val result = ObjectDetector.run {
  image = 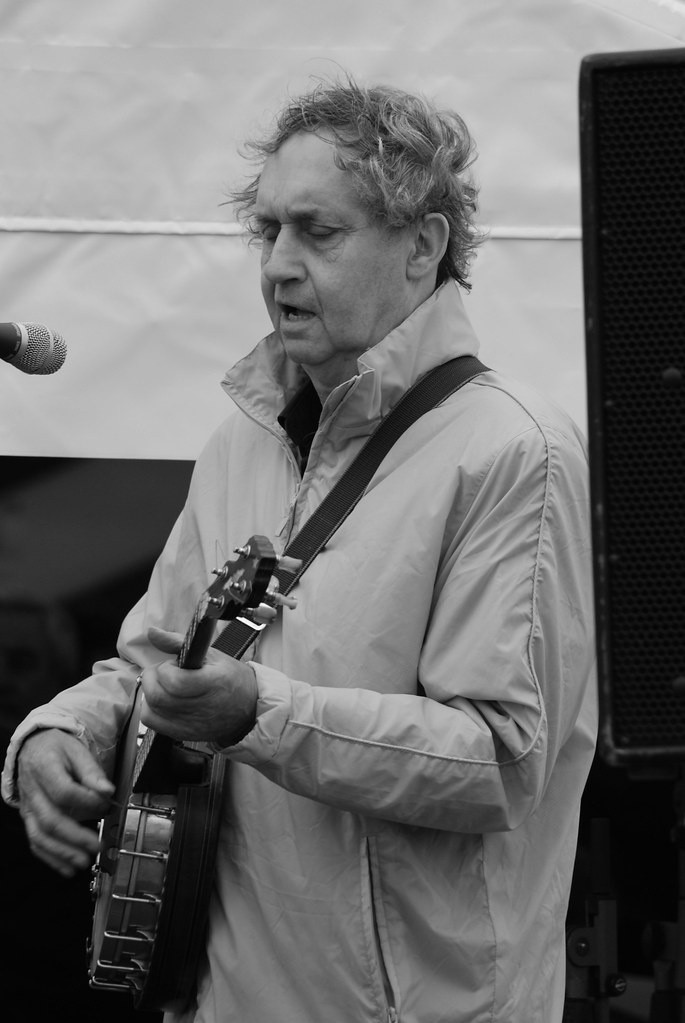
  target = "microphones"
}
[0,322,68,375]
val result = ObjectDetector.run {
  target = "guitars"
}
[86,536,302,1010]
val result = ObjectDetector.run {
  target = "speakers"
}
[579,48,685,769]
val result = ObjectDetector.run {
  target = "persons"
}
[1,70,601,1022]
